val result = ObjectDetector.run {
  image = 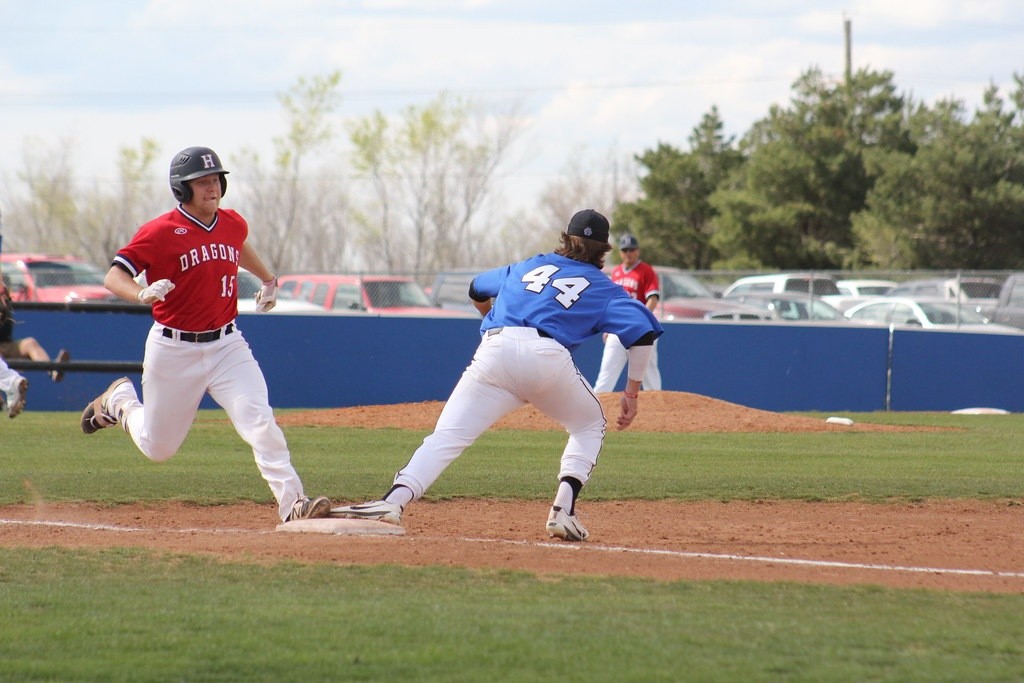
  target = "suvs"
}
[1,252,124,302]
[277,275,469,319]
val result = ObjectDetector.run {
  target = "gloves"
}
[255,275,277,312]
[138,279,176,305]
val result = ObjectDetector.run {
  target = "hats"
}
[618,234,639,249]
[567,209,610,243]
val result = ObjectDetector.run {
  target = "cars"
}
[133,265,326,312]
[724,273,1024,333]
[601,263,783,320]
[431,269,492,318]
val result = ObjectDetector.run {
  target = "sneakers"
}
[329,499,403,526]
[287,496,331,521]
[7,377,28,419]
[52,350,68,382]
[80,376,133,434]
[545,506,588,540]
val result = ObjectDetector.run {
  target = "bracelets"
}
[260,275,276,286]
[623,390,638,398]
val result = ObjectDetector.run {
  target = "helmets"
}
[169,146,230,203]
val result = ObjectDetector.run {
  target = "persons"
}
[0,282,68,418]
[81,147,332,522]
[327,209,664,545]
[593,232,662,393]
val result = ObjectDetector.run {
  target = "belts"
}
[160,324,234,343]
